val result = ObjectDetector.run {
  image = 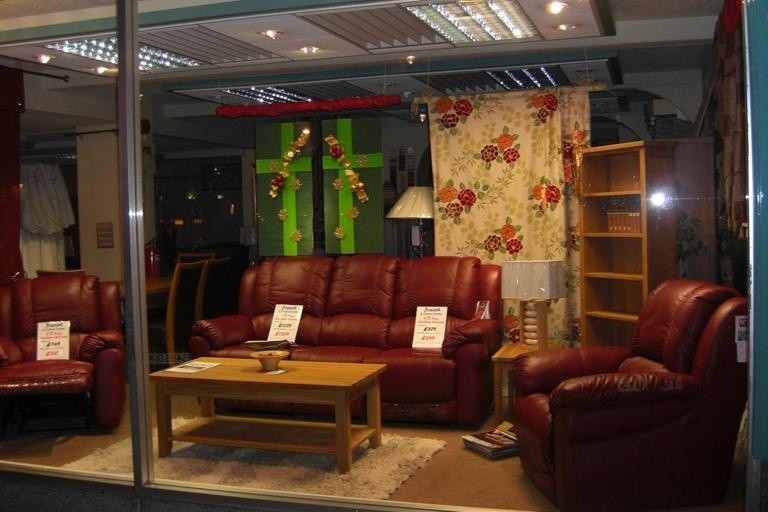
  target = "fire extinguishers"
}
[145,235,160,282]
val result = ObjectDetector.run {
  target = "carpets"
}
[57,415,448,502]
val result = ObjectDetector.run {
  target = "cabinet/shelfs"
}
[578,138,679,347]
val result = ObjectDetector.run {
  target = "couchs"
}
[187,254,505,431]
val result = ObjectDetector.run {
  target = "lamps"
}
[500,260,567,347]
[383,185,434,257]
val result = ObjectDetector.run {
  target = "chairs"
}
[509,276,749,512]
[148,259,210,369]
[176,252,216,262]
[0,271,127,431]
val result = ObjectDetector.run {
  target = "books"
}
[606,202,641,232]
[462,426,519,461]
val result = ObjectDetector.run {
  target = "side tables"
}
[118,275,172,351]
[490,341,561,418]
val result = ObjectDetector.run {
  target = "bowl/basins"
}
[249,350,289,371]
